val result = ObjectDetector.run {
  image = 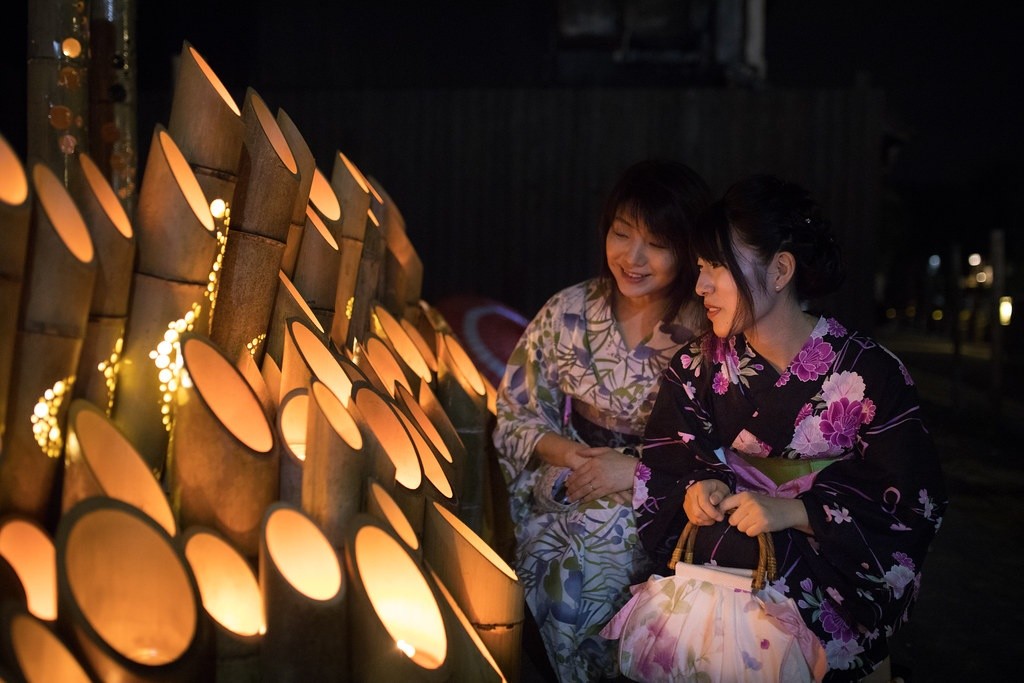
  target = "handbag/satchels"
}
[597,503,830,683]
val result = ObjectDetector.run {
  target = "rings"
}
[589,482,596,491]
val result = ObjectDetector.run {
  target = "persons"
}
[633,172,949,683]
[491,158,713,683]
